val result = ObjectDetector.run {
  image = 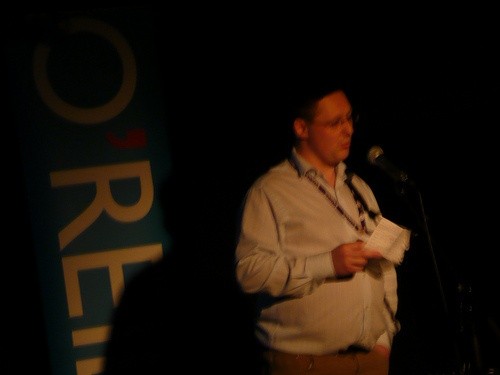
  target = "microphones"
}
[367,146,417,189]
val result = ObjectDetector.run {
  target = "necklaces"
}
[287,152,374,237]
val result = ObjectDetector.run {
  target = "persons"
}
[236,85,400,375]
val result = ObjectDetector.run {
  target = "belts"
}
[338,344,369,355]
